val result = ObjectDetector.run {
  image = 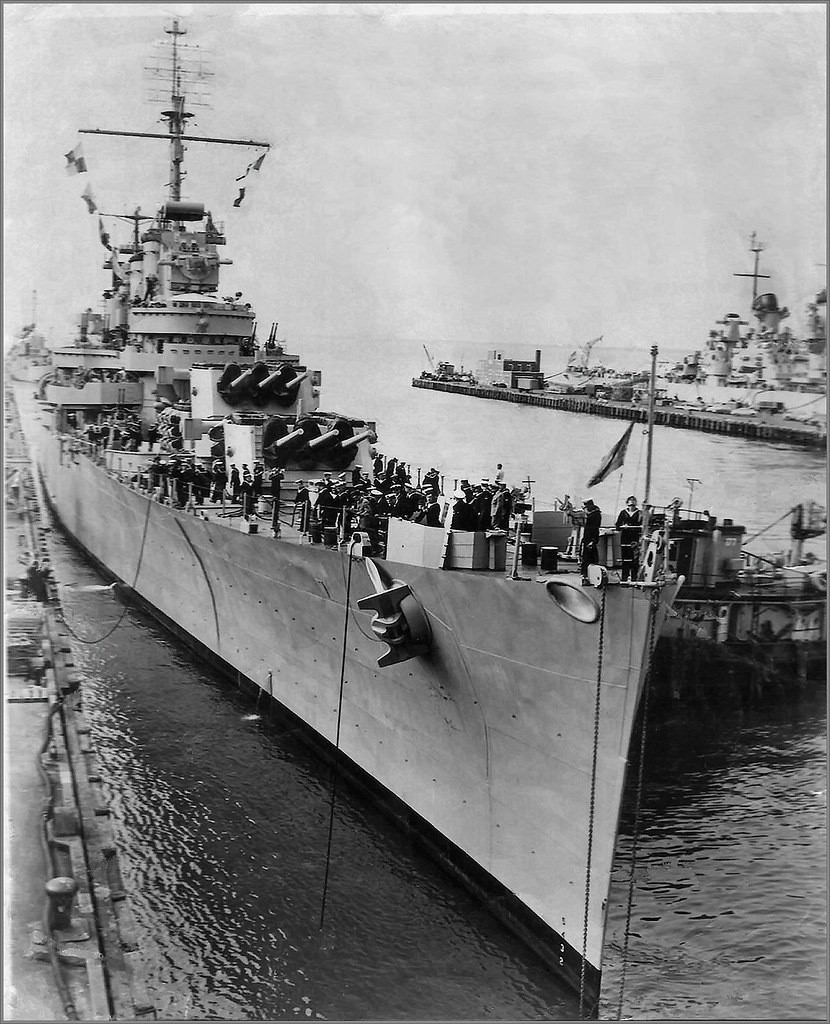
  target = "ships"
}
[1,19,692,1023]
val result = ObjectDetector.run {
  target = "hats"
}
[88,415,140,435]
[148,453,280,478]
[583,499,594,505]
[379,453,406,464]
[292,464,439,499]
[461,478,507,491]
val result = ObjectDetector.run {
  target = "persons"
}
[83,412,159,452]
[293,454,445,551]
[450,464,512,537]
[143,276,155,302]
[580,501,602,587]
[144,453,284,520]
[615,497,644,582]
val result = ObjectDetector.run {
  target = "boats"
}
[635,233,829,420]
[549,360,638,394]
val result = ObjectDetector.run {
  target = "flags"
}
[64,150,114,251]
[587,415,638,488]
[233,154,266,208]
